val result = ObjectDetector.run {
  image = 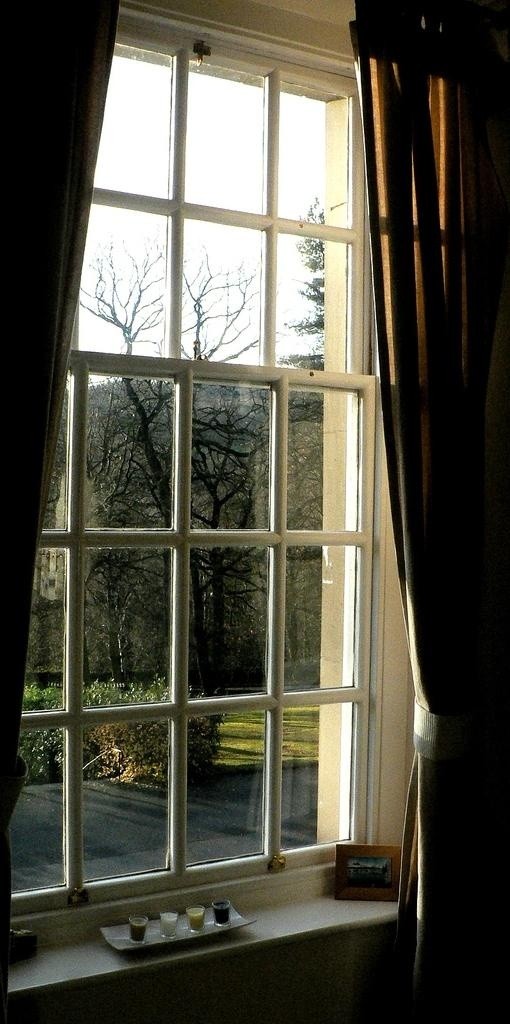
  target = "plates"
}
[98,904,258,952]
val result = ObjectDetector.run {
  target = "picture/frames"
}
[334,842,402,902]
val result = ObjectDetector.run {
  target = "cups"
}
[128,914,149,944]
[159,910,179,938]
[211,898,232,928]
[185,904,207,933]
